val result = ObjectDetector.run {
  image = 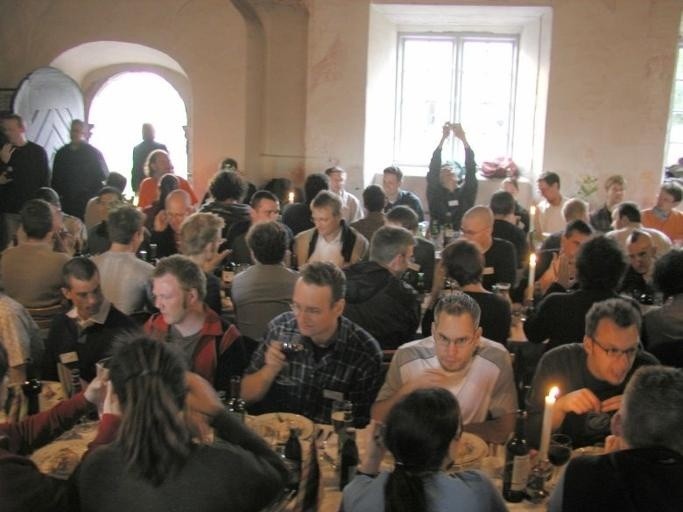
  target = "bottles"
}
[284,430,302,489]
[221,253,235,298]
[149,244,157,265]
[339,429,360,490]
[430,209,440,250]
[69,368,82,398]
[20,357,43,418]
[533,281,544,307]
[226,376,246,425]
[502,409,532,503]
[289,252,299,272]
[442,212,453,248]
[415,273,425,303]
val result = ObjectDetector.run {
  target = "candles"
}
[287,192,295,204]
[527,254,537,299]
[539,396,557,462]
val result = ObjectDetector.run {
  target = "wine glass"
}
[277,333,303,386]
[331,400,353,433]
[547,434,573,490]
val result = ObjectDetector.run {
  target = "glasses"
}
[590,336,644,360]
[457,227,489,238]
[406,254,417,266]
[285,300,324,318]
[654,192,672,205]
[433,328,477,348]
[625,250,648,265]
[308,217,329,224]
[164,209,187,221]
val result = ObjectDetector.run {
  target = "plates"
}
[252,411,314,445]
[458,431,487,463]
[33,439,85,479]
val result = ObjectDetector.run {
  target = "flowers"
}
[574,174,598,195]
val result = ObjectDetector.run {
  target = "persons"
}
[528,171,571,242]
[426,121,478,232]
[382,167,425,223]
[325,166,364,223]
[72,335,293,512]
[200,158,258,205]
[281,172,330,237]
[517,219,592,304]
[461,206,517,293]
[142,255,250,402]
[86,186,151,254]
[0,287,48,393]
[0,198,73,323]
[524,300,661,455]
[50,119,110,222]
[339,386,510,512]
[421,240,512,348]
[616,228,661,304]
[641,182,683,243]
[0,114,50,217]
[85,208,157,323]
[150,189,195,254]
[44,257,138,385]
[228,190,296,268]
[489,191,530,269]
[589,175,627,233]
[640,249,682,367]
[384,205,435,293]
[197,170,256,259]
[546,366,683,512]
[175,212,233,315]
[84,172,127,239]
[340,225,422,349]
[603,202,673,264]
[370,293,518,446]
[230,223,302,342]
[137,149,199,208]
[265,177,295,224]
[240,261,384,429]
[349,184,389,241]
[523,235,642,350]
[0,362,124,512]
[539,198,591,263]
[141,174,180,229]
[131,123,170,197]
[293,191,369,275]
[5,186,83,261]
[493,176,529,235]
